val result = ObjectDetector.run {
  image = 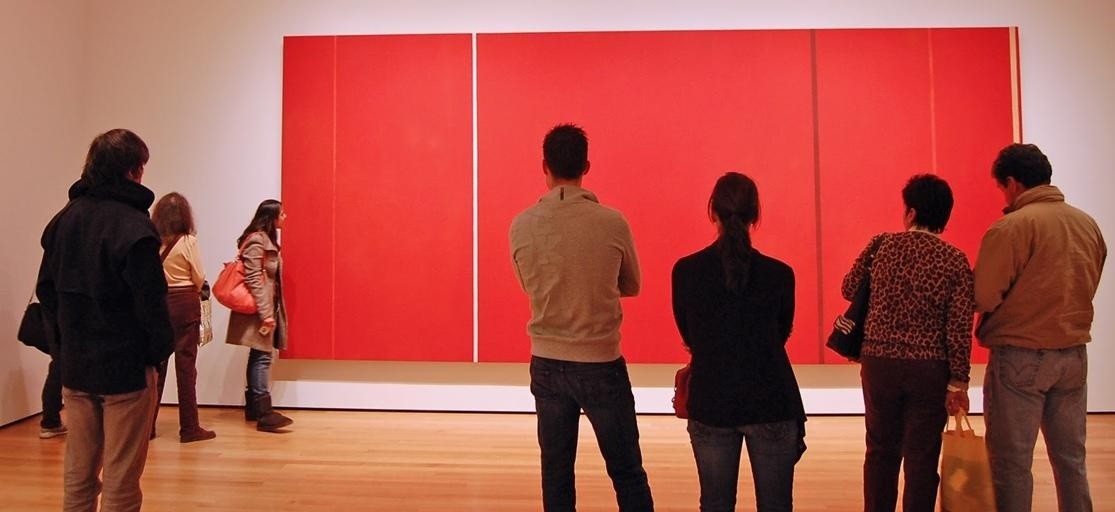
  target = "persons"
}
[971,142,1109,512]
[505,120,656,511]
[224,198,293,431]
[672,172,810,511]
[149,191,216,445]
[33,128,175,512]
[38,357,69,440]
[841,172,976,512]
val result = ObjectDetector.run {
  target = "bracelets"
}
[946,384,968,393]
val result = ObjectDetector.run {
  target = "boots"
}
[255,394,293,430]
[244,391,282,421]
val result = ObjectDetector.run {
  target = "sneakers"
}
[39,426,67,439]
[180,428,215,442]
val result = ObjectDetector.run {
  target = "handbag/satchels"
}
[939,408,997,512]
[671,366,689,419]
[198,299,213,347]
[826,234,880,362]
[213,231,268,315]
[18,301,51,353]
